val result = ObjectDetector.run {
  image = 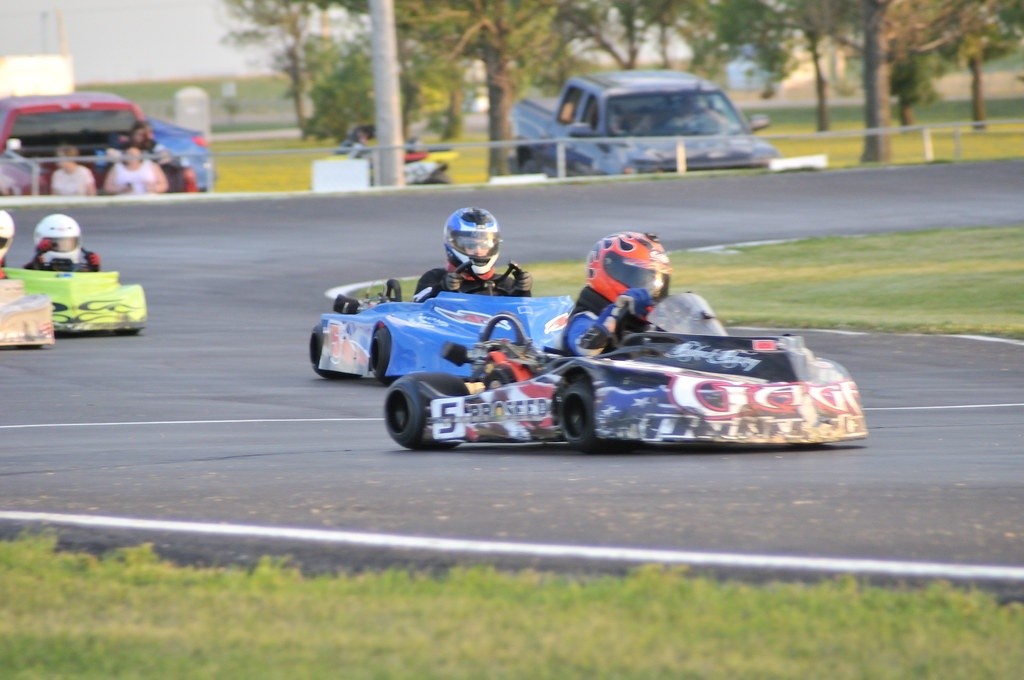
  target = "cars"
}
[0,91,215,194]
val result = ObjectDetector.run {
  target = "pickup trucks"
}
[512,68,780,175]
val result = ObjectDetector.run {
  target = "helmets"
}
[587,232,671,323]
[0,210,14,261]
[443,207,501,274]
[33,213,81,264]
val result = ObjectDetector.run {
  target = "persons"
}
[105,121,171,166]
[562,232,699,362]
[23,213,100,271]
[101,146,169,195]
[50,144,96,196]
[335,129,372,159]
[413,206,533,303]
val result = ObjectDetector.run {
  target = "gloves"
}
[440,272,462,290]
[614,288,655,315]
[512,271,532,291]
